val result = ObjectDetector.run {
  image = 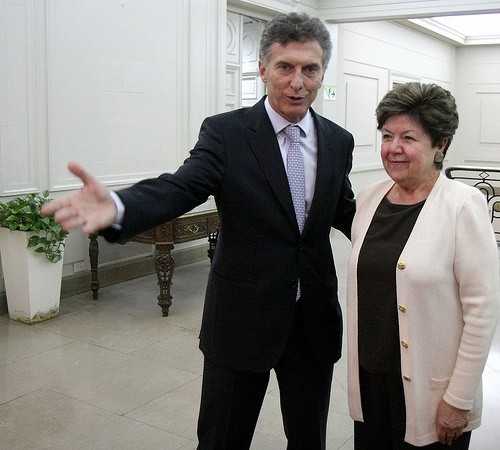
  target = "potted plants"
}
[0,186,72,327]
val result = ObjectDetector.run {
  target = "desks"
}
[88,194,222,317]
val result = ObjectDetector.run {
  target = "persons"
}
[40,12,357,450]
[346,82,500,450]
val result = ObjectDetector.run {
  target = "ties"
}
[281,126,306,305]
